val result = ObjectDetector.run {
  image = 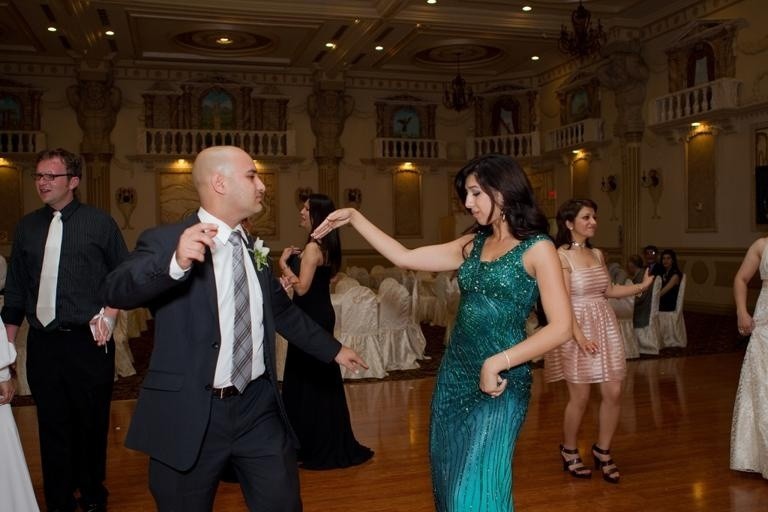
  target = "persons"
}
[278,195,374,471]
[656,249,682,311]
[543,197,655,486]
[642,244,663,276]
[726,235,767,481]
[626,252,653,328]
[0,147,129,511]
[0,308,42,511]
[97,146,367,512]
[310,153,572,512]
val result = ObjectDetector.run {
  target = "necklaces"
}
[570,243,589,248]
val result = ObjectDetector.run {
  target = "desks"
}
[13,307,153,396]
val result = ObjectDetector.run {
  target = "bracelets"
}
[634,282,642,298]
[502,351,511,371]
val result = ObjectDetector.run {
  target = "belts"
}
[211,386,244,396]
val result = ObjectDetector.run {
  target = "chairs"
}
[658,273,688,349]
[433,273,449,327]
[613,279,641,362]
[635,275,663,355]
[444,277,462,345]
[330,258,643,295]
[334,285,389,379]
[526,310,546,362]
[416,268,435,324]
[406,280,433,360]
[376,283,421,371]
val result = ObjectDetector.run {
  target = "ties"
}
[228,231,252,395]
[36,211,63,328]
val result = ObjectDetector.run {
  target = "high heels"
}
[591,443,620,482]
[559,444,592,477]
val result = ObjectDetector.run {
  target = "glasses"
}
[32,172,71,182]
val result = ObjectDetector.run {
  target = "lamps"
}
[441,54,475,111]
[299,188,311,203]
[118,187,134,203]
[348,189,357,202]
[641,169,659,189]
[600,175,617,193]
[558,0,607,65]
